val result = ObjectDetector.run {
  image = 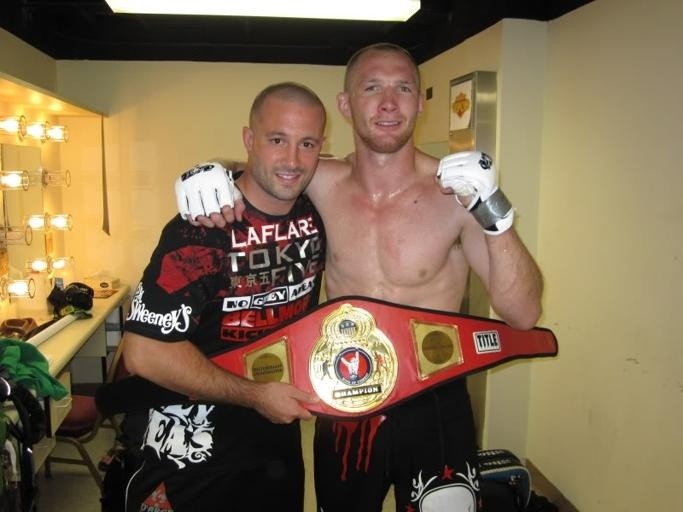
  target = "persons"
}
[99,80,326,510]
[172,42,546,512]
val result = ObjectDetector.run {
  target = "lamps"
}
[103,0,422,23]
[0,114,74,304]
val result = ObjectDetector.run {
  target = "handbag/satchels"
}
[477,449,531,508]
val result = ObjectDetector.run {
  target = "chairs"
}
[45,330,126,494]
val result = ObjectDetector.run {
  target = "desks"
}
[0,285,132,512]
